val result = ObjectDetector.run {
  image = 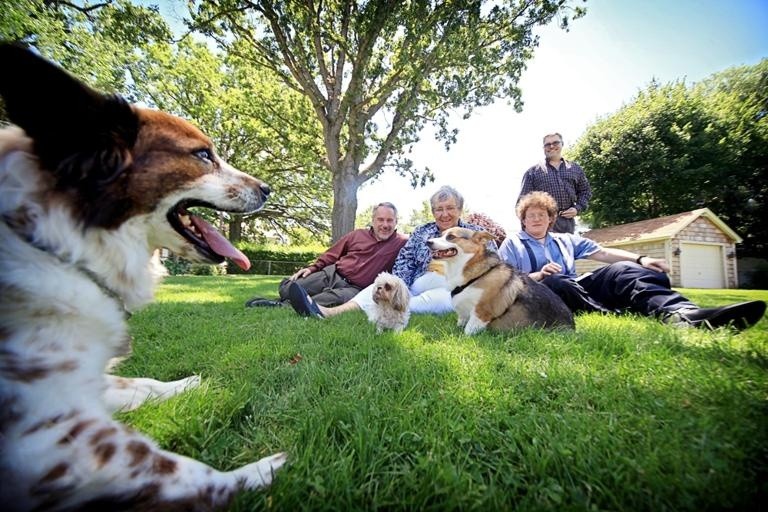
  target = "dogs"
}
[0,39,288,512]
[366,270,411,336]
[426,227,576,337]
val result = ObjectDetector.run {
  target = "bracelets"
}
[636,255,646,264]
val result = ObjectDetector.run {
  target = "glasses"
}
[544,141,561,148]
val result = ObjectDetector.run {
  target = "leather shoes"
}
[663,300,766,336]
[247,282,325,320]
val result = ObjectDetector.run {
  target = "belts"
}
[335,271,352,284]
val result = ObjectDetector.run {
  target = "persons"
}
[496,191,766,335]
[247,201,409,309]
[516,133,591,234]
[289,185,499,320]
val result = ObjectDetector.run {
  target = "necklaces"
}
[531,236,546,240]
